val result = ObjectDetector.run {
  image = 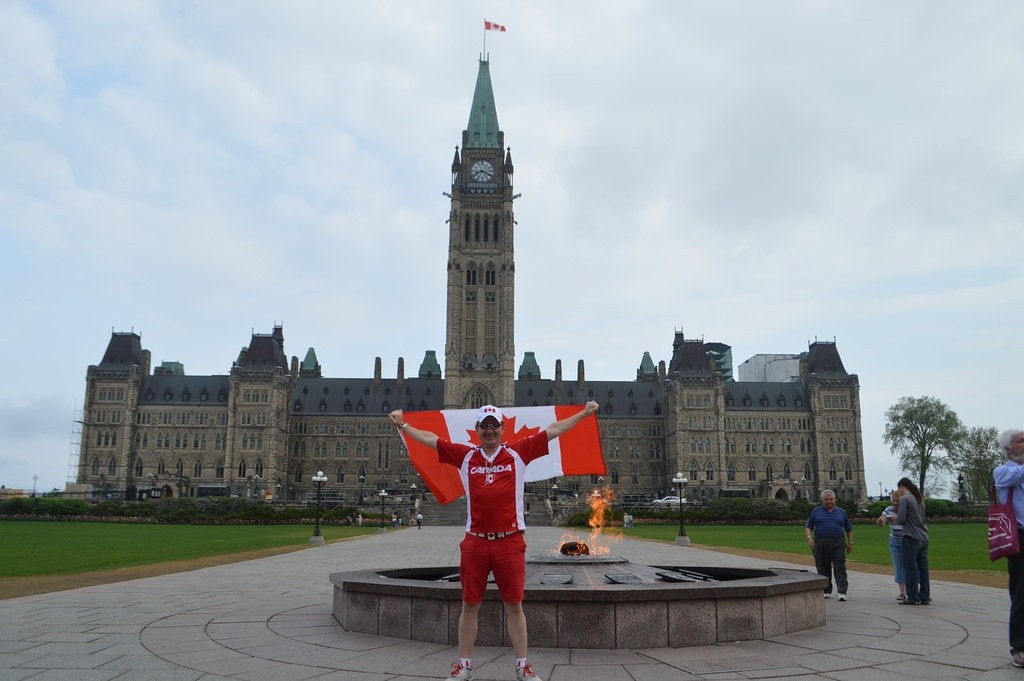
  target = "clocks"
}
[470,159,496,184]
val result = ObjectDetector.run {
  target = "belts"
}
[467,530,516,540]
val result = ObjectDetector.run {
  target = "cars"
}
[550,487,580,498]
[652,496,688,504]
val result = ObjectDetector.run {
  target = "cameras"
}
[886,515,892,519]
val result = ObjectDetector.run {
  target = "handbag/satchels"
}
[987,479,1020,562]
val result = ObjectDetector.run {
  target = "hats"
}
[478,405,503,423]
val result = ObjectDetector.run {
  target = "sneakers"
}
[515,663,542,681]
[445,662,474,681]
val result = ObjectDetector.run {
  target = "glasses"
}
[477,423,501,430]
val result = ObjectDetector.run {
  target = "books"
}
[886,515,893,523]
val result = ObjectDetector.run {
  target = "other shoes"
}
[897,594,906,600]
[1009,651,1024,667]
[838,593,847,601]
[823,593,829,598]
[919,600,929,605]
[899,598,920,605]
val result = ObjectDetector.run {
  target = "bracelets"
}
[398,421,409,431]
[807,535,811,539]
[848,541,854,545]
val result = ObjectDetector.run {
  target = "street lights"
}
[379,490,388,529]
[32,474,39,497]
[557,480,561,495]
[800,476,807,496]
[672,473,688,537]
[409,483,417,518]
[311,471,328,536]
[359,475,366,499]
[147,473,153,490]
[253,475,259,495]
[878,481,884,501]
[277,484,281,500]
[599,476,604,492]
[417,472,421,492]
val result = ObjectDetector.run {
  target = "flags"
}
[393,404,608,507]
[485,21,506,32]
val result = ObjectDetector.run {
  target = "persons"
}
[260,487,270,501]
[398,516,402,527]
[805,489,854,601]
[993,427,1024,667]
[876,492,913,600]
[392,512,397,527]
[387,400,600,681]
[892,477,930,605]
[958,474,966,489]
[417,512,423,529]
